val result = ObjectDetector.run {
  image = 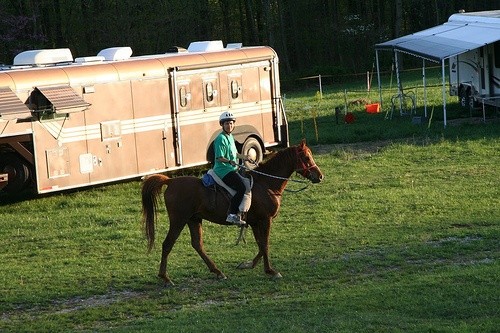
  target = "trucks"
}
[0,38,289,194]
[448,9,500,113]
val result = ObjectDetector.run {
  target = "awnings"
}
[374,22,500,128]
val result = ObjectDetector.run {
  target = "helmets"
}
[219,112,235,124]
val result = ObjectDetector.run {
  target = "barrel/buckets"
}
[364,102,380,113]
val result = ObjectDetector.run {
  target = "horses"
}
[140,137,325,290]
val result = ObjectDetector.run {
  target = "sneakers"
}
[226,215,246,224]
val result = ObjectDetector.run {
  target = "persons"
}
[213,112,251,225]
